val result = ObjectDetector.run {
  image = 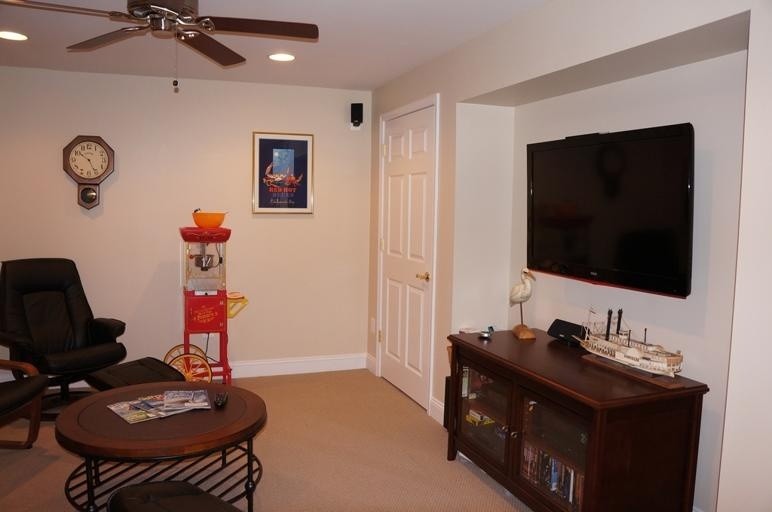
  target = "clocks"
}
[62,135,115,210]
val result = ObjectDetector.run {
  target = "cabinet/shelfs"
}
[446,328,709,512]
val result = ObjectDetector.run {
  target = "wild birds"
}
[509,267,536,307]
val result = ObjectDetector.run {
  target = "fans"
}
[0,0,318,66]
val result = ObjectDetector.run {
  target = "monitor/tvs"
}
[526,123,694,298]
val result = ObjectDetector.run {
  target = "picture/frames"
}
[252,131,314,215]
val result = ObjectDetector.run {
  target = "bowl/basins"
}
[191,211,227,228]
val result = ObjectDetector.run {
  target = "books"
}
[465,408,584,510]
[163,390,195,410]
[185,389,214,410]
[105,400,150,426]
[129,402,164,420]
[137,394,181,412]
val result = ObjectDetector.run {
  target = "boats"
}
[572,304,684,378]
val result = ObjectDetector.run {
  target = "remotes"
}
[214,393,227,407]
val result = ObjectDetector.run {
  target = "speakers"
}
[351,103,363,126]
[547,319,591,344]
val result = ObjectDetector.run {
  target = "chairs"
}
[0,258,186,449]
